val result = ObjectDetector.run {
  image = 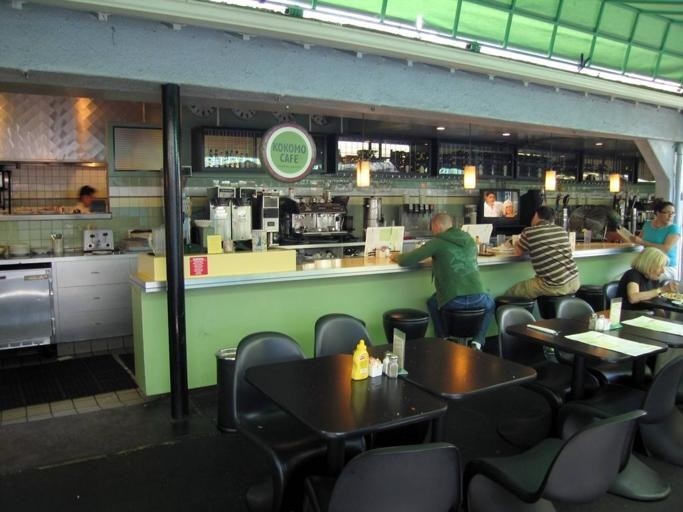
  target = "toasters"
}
[82,228,116,253]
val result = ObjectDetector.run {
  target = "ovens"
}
[0,262,57,369]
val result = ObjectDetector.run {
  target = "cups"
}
[583,230,591,243]
[568,231,576,249]
[223,239,234,252]
[497,234,506,246]
[52,239,63,255]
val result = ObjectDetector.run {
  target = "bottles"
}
[350,339,399,381]
[350,378,368,424]
[322,191,330,203]
[287,188,294,200]
[442,144,637,185]
[588,313,610,332]
[205,149,253,169]
[474,235,487,254]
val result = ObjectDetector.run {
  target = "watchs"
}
[655,287,662,297]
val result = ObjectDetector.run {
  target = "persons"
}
[615,247,679,371]
[73,185,97,214]
[505,205,581,301]
[484,191,500,217]
[629,201,681,318]
[502,199,517,218]
[389,212,496,351]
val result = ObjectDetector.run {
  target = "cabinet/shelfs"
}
[190,126,335,174]
[51,258,136,342]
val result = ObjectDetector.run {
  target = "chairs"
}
[302,444,463,511]
[552,296,653,388]
[464,403,646,511]
[604,283,619,311]
[313,313,434,444]
[231,332,365,510]
[495,304,598,404]
[567,357,679,442]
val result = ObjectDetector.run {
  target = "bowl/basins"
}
[194,219,211,227]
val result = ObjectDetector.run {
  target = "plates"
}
[8,243,31,257]
[29,247,49,257]
[660,293,683,302]
[492,247,515,251]
[15,210,34,215]
[36,210,58,215]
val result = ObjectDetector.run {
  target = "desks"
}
[245,354,449,469]
[505,317,668,399]
[572,307,682,388]
[640,296,682,314]
[351,336,538,444]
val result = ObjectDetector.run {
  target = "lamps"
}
[464,122,476,189]
[356,115,370,187]
[609,139,621,192]
[544,132,556,190]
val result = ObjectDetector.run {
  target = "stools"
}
[579,285,604,301]
[445,306,485,342]
[493,295,536,325]
[383,308,429,343]
[538,293,574,318]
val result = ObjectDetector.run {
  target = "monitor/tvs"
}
[461,224,493,253]
[480,188,521,225]
[363,226,404,257]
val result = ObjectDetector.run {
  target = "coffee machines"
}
[280,194,356,244]
[617,199,656,236]
